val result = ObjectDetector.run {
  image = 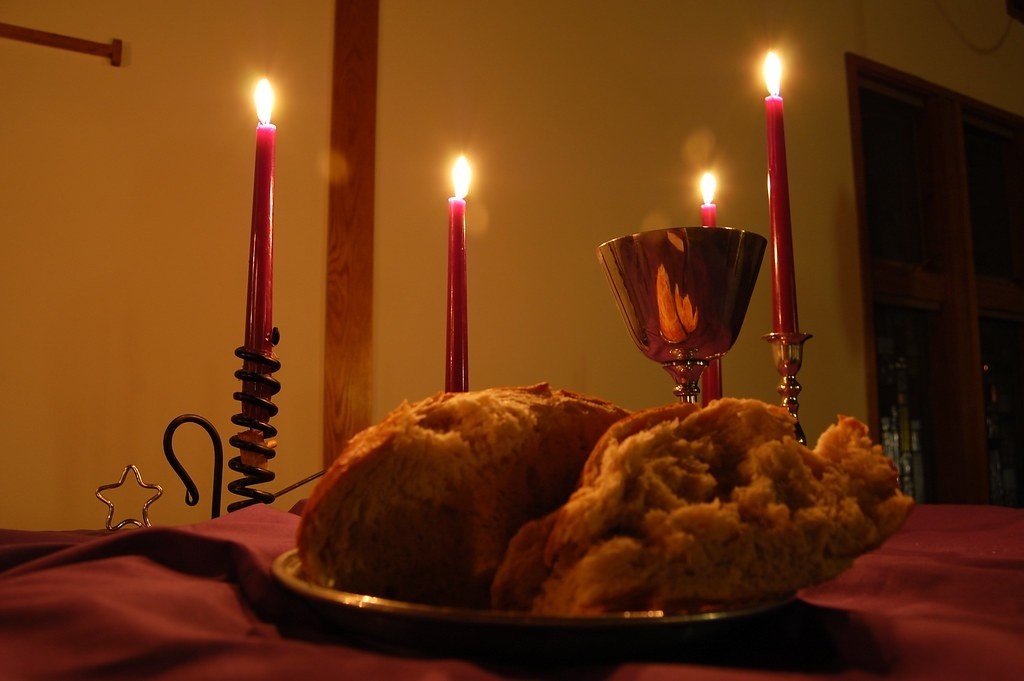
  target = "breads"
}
[297,382,643,601]
[490,393,913,612]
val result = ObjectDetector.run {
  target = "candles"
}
[445,197,469,395]
[702,204,722,409]
[237,124,276,471]
[764,94,798,334]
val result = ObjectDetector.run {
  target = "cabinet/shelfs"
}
[844,51,1024,508]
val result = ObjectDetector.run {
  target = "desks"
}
[0,502,1024,681]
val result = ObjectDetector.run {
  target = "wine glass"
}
[594,226,769,405]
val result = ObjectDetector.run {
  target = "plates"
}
[270,548,796,632]
